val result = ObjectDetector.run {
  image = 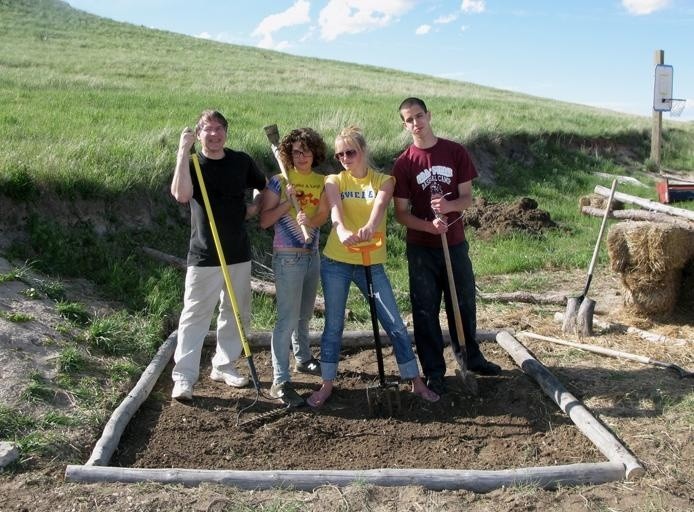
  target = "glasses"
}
[291,149,313,158]
[334,149,357,160]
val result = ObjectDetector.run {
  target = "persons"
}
[259,128,330,406]
[391,98,502,395]
[171,109,267,402]
[306,123,440,407]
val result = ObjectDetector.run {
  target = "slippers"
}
[411,388,440,402]
[306,390,333,407]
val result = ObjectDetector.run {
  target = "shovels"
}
[562,178,617,337]
[441,233,484,397]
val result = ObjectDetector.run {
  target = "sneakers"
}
[210,364,249,387]
[295,355,322,377]
[427,372,444,394]
[270,380,305,406]
[468,355,501,376]
[171,380,194,400]
